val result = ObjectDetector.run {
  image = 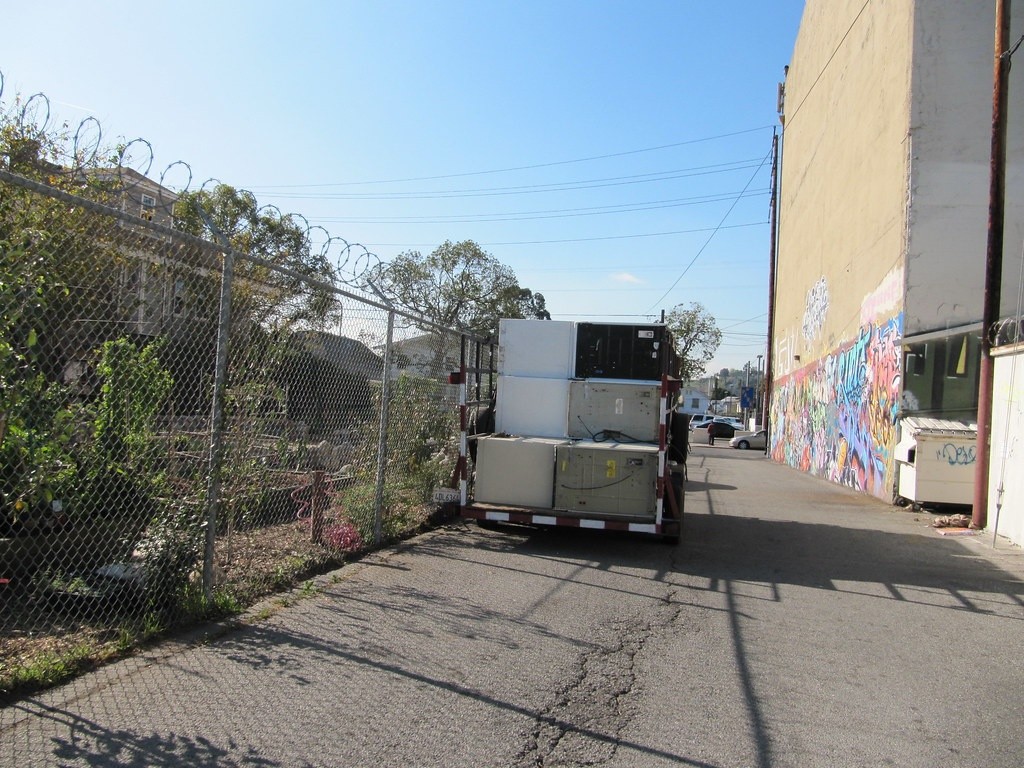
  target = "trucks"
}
[462,310,688,547]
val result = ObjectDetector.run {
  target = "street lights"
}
[756,354,764,424]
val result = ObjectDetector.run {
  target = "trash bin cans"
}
[894,416,977,505]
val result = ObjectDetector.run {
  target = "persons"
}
[707,421,716,446]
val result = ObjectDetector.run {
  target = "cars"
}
[729,428,767,450]
[689,414,744,438]
